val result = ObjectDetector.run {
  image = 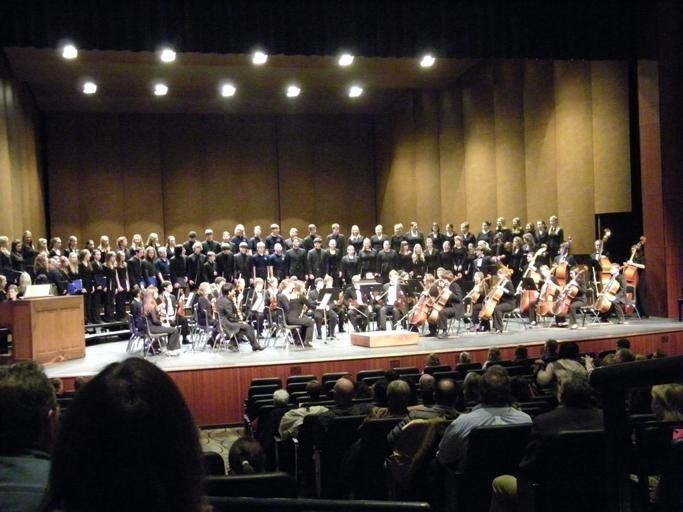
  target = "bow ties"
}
[596,252,599,254]
[478,256,483,259]
[389,284,394,286]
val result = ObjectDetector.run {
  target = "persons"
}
[547,216,563,261]
[102,252,118,320]
[400,273,414,298]
[404,221,425,251]
[630,245,651,320]
[276,279,291,324]
[234,278,251,309]
[615,338,630,353]
[326,240,343,288]
[489,376,616,511]
[142,249,158,289]
[425,355,440,367]
[187,242,207,290]
[324,276,346,332]
[444,224,457,249]
[650,382,683,424]
[130,234,144,255]
[307,277,338,340]
[0,235,15,284]
[553,242,576,270]
[424,238,439,275]
[48,237,64,258]
[62,235,81,257]
[452,235,467,275]
[79,249,96,324]
[510,217,525,239]
[258,389,297,450]
[6,285,21,302]
[228,436,265,476]
[233,242,256,287]
[251,242,272,285]
[358,238,377,278]
[146,232,160,255]
[128,248,144,289]
[511,236,523,286]
[460,223,476,249]
[347,224,365,255]
[534,342,589,405]
[0,357,63,512]
[217,231,236,255]
[384,378,462,475]
[21,229,39,278]
[156,281,192,344]
[478,240,495,258]
[43,358,213,511]
[473,246,492,281]
[476,269,517,335]
[466,242,476,270]
[362,380,411,421]
[326,224,346,254]
[590,241,604,263]
[98,235,110,263]
[201,229,221,256]
[307,238,330,282]
[462,272,487,331]
[529,264,568,326]
[266,279,279,322]
[377,270,400,330]
[371,224,386,251]
[439,241,454,271]
[344,274,370,332]
[384,369,400,383]
[424,270,465,339]
[131,288,161,355]
[537,221,548,244]
[250,226,267,253]
[557,267,587,329]
[36,274,48,286]
[287,280,316,347]
[208,277,227,302]
[143,285,180,356]
[169,245,191,297]
[531,340,560,371]
[427,223,445,253]
[513,346,529,364]
[596,262,626,324]
[42,256,72,294]
[408,273,436,332]
[247,278,270,339]
[266,224,287,255]
[115,251,131,317]
[19,274,31,296]
[461,372,486,416]
[0,276,9,303]
[355,381,371,398]
[476,222,494,248]
[521,253,545,295]
[69,252,87,295]
[340,245,361,285]
[375,238,400,285]
[231,223,250,253]
[503,241,513,263]
[482,347,502,372]
[202,252,218,283]
[584,349,636,379]
[525,222,537,243]
[197,282,223,349]
[392,223,407,253]
[182,231,201,255]
[403,377,419,408]
[652,350,667,361]
[166,236,177,259]
[115,236,132,259]
[436,267,447,280]
[511,377,552,421]
[412,244,428,277]
[49,379,64,397]
[216,243,234,283]
[285,227,304,251]
[399,241,413,272]
[10,240,24,272]
[303,224,323,251]
[286,237,308,282]
[521,233,538,264]
[215,283,266,351]
[420,371,531,512]
[315,377,375,451]
[495,217,512,242]
[269,243,287,285]
[459,352,472,365]
[156,246,171,285]
[85,240,96,252]
[37,238,50,255]
[90,249,107,320]
[305,380,322,402]
[360,380,389,416]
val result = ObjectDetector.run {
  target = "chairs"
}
[276,307,305,349]
[393,367,423,382]
[264,306,276,347]
[447,297,478,336]
[626,286,642,322]
[521,357,543,375]
[628,414,683,511]
[580,289,601,328]
[468,423,545,474]
[322,371,353,405]
[244,376,295,443]
[457,362,486,377]
[486,360,523,377]
[286,375,335,407]
[203,473,296,496]
[598,350,617,360]
[357,369,386,388]
[578,351,596,361]
[363,418,405,450]
[501,293,528,332]
[190,302,214,352]
[302,414,366,453]
[423,365,458,381]
[213,311,240,352]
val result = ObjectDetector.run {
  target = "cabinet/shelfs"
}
[8,295,86,364]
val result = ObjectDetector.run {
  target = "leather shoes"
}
[166,351,180,357]
[228,344,239,353]
[493,329,504,334]
[207,338,216,348]
[426,331,438,337]
[529,322,538,328]
[316,335,323,340]
[304,341,313,347]
[466,322,471,329]
[182,339,193,344]
[148,348,160,355]
[340,330,347,332]
[253,346,267,352]
[271,332,276,337]
[295,339,303,345]
[257,334,265,339]
[328,334,337,340]
[570,324,579,330]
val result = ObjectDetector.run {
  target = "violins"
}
[177,290,186,316]
[338,290,368,320]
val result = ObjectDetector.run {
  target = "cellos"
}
[592,266,623,313]
[555,234,574,285]
[478,261,509,321]
[515,243,547,315]
[535,263,557,316]
[462,272,491,303]
[408,292,436,327]
[549,266,588,316]
[597,227,613,291]
[420,273,462,325]
[623,235,648,317]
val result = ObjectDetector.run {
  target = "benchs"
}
[589,355,683,386]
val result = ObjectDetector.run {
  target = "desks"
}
[126,311,169,359]
